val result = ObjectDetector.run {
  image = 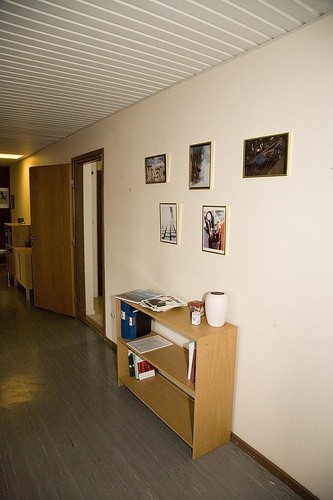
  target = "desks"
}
[13,246,33,302]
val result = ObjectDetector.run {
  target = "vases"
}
[204,291,228,327]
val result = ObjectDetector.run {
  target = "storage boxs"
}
[188,300,205,316]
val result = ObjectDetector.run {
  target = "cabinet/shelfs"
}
[115,294,237,460]
[5,223,31,288]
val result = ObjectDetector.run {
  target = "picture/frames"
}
[201,205,226,255]
[243,132,289,178]
[188,141,212,190]
[159,203,178,245]
[144,154,167,184]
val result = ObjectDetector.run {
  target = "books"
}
[188,341,196,383]
[128,349,156,380]
[127,335,174,355]
[114,288,188,340]
